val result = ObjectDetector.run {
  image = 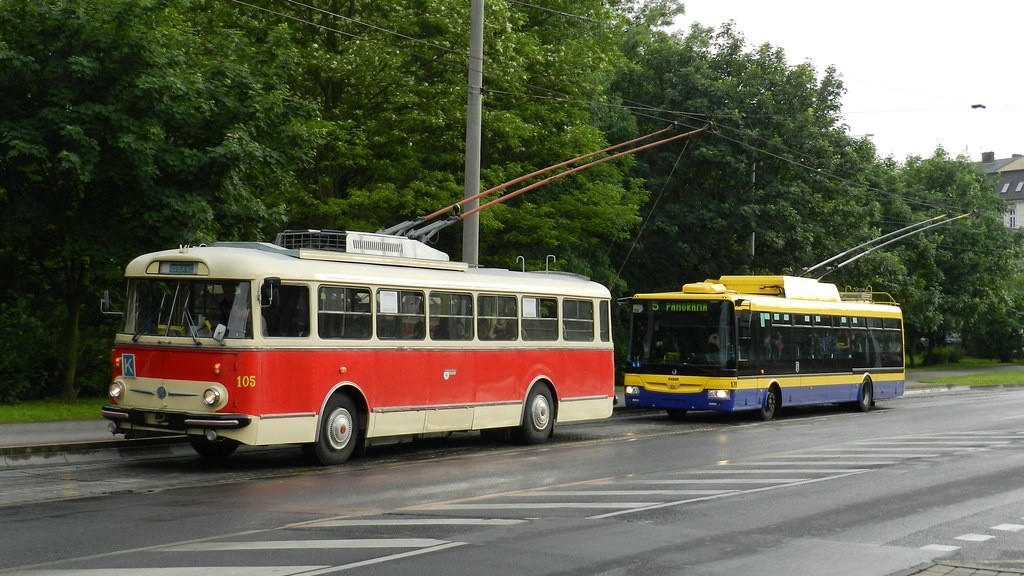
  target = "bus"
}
[615,211,972,422]
[100,120,714,467]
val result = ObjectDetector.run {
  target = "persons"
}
[686,334,731,366]
[411,311,527,339]
[223,293,268,338]
[801,331,901,369]
